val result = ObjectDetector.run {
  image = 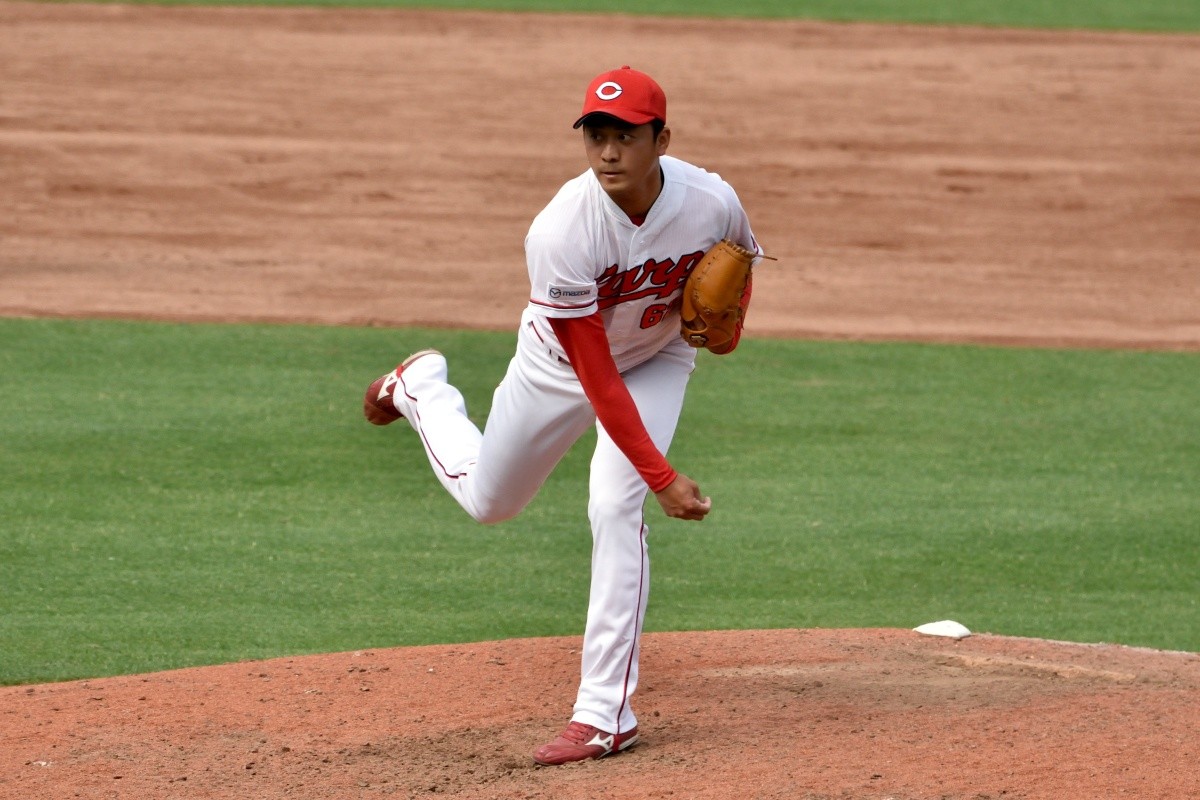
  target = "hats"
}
[573,65,667,128]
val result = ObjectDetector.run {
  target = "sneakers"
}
[362,348,443,425]
[532,720,640,767]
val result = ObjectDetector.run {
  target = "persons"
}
[365,65,765,768]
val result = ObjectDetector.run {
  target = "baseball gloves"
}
[680,238,756,348]
